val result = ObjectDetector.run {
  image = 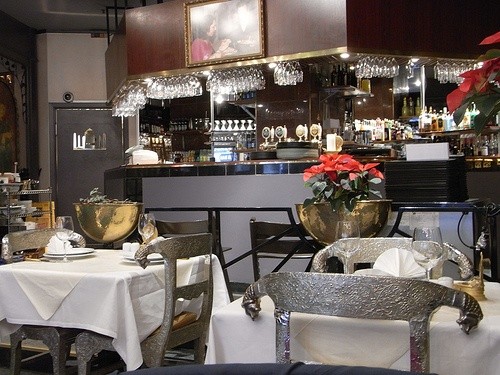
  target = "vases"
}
[295,200,395,245]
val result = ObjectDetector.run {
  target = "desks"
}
[204,279,500,374]
[0,249,230,375]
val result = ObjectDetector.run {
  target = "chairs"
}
[0,217,483,375]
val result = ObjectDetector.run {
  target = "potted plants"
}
[72,187,144,244]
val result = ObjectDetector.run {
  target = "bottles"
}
[340,97,500,157]
[139,118,257,163]
[328,63,362,89]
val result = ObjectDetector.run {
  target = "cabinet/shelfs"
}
[137,72,395,158]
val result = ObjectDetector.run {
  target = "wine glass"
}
[110,73,203,118]
[334,222,361,274]
[434,58,483,85]
[355,54,415,79]
[137,212,156,243]
[55,215,74,264]
[206,65,266,93]
[410,227,445,281]
[273,60,305,86]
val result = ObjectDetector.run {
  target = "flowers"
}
[445,31,500,136]
[302,152,385,215]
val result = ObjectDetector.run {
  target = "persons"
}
[191,15,232,61]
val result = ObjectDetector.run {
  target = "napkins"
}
[49,232,73,251]
[373,248,427,277]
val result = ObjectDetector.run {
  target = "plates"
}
[249,142,318,160]
[43,247,164,264]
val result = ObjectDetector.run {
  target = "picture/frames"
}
[183,0,266,69]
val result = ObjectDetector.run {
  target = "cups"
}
[122,242,140,255]
[439,277,454,288]
